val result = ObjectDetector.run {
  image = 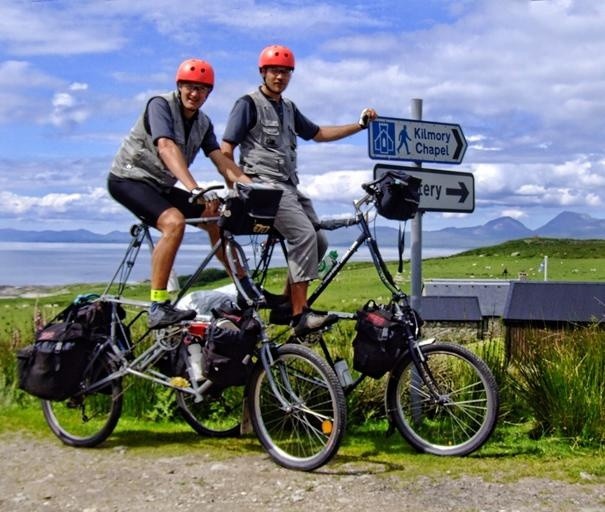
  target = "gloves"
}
[358,108,373,129]
[190,187,220,202]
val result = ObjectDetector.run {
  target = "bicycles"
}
[175,172,500,456]
[40,186,347,468]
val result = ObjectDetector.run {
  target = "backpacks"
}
[16,300,88,402]
[59,292,137,395]
[351,299,421,380]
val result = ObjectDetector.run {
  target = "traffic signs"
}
[373,164,475,213]
[367,117,467,165]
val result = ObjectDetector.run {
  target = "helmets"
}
[258,44,297,71]
[174,57,215,92]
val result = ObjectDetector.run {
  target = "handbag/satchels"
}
[201,298,263,387]
[216,182,285,235]
[372,170,424,220]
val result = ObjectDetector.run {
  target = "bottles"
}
[187,337,210,382]
[334,356,353,387]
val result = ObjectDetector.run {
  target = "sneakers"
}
[236,290,292,311]
[292,311,340,339]
[146,300,197,329]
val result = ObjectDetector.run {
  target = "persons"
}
[106,57,292,328]
[218,44,378,336]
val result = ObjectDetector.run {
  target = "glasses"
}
[180,82,210,93]
